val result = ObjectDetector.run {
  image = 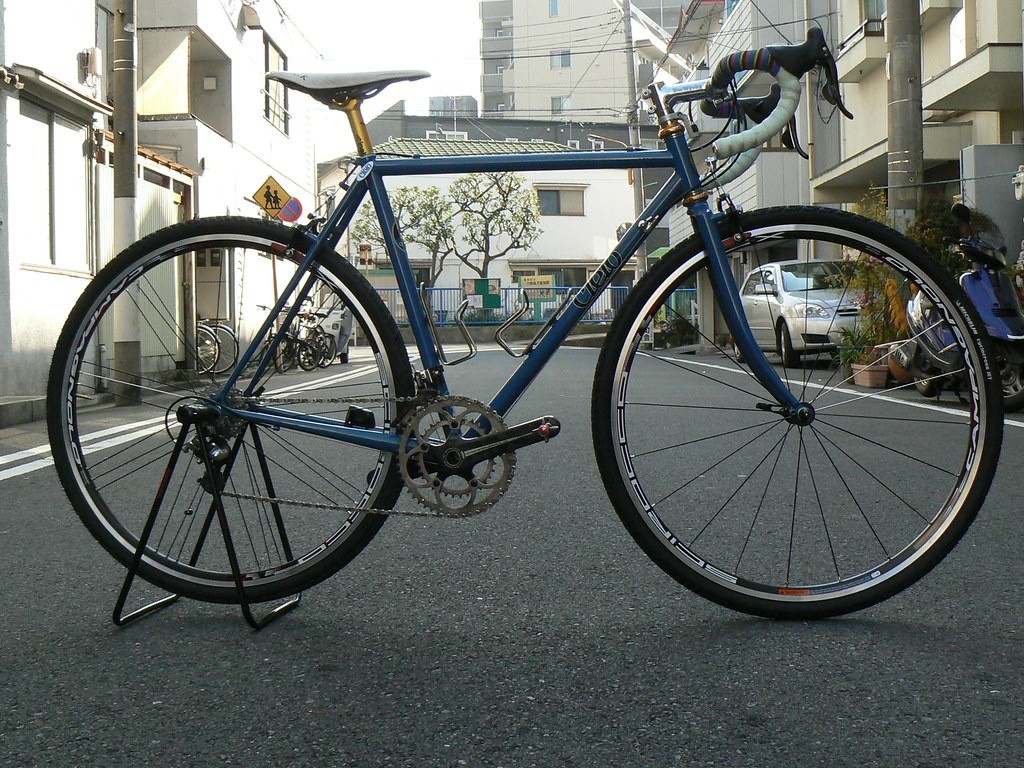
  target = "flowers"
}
[823,192,898,346]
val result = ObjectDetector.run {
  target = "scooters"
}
[898,202,1024,408]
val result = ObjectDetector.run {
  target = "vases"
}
[863,345,875,354]
[888,347,913,381]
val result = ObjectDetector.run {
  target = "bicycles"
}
[257,296,336,374]
[195,317,240,375]
[46,28,1005,631]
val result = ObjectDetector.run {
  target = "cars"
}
[732,259,874,369]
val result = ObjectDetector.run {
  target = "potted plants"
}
[715,335,728,347]
[851,347,891,389]
[830,324,870,383]
[663,311,699,349]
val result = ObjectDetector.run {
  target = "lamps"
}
[82,46,103,78]
